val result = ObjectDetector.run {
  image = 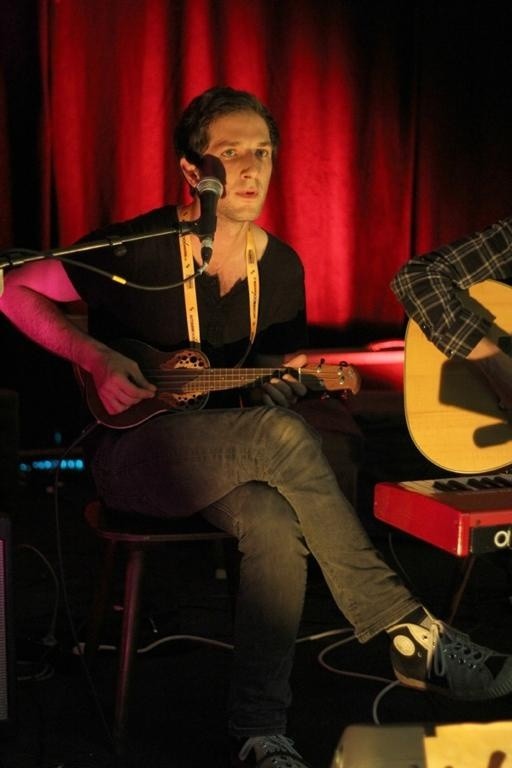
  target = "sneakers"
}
[386,606,512,701]
[238,734,308,768]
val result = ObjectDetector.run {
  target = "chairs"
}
[64,315,243,754]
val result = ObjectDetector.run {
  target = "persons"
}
[2,83,512,766]
[388,203,511,434]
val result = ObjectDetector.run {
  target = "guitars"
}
[75,338,359,431]
[403,280,512,474]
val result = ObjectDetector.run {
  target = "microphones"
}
[197,176,223,265]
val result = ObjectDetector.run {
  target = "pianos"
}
[370,473,510,560]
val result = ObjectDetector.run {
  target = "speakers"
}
[329,724,512,768]
